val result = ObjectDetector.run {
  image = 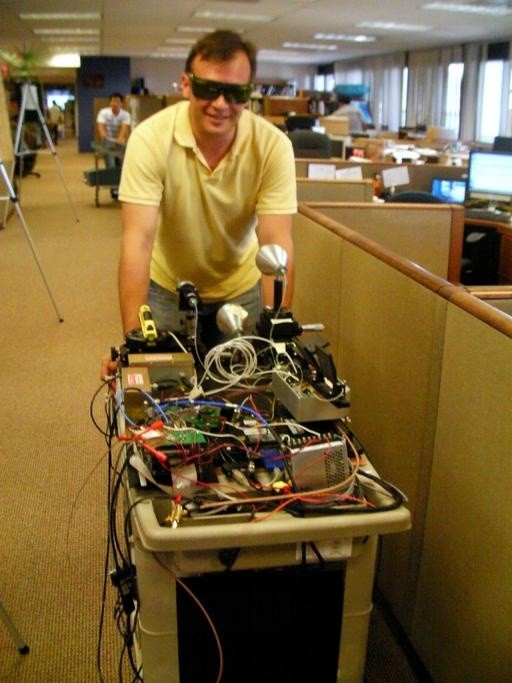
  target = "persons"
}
[96,90,134,199]
[99,26,299,402]
[48,101,64,145]
[329,94,369,132]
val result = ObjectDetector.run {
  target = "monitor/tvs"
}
[430,178,467,204]
[466,149,512,214]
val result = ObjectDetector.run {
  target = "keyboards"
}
[464,210,510,222]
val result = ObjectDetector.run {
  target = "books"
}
[245,82,297,116]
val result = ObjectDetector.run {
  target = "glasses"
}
[187,70,254,104]
